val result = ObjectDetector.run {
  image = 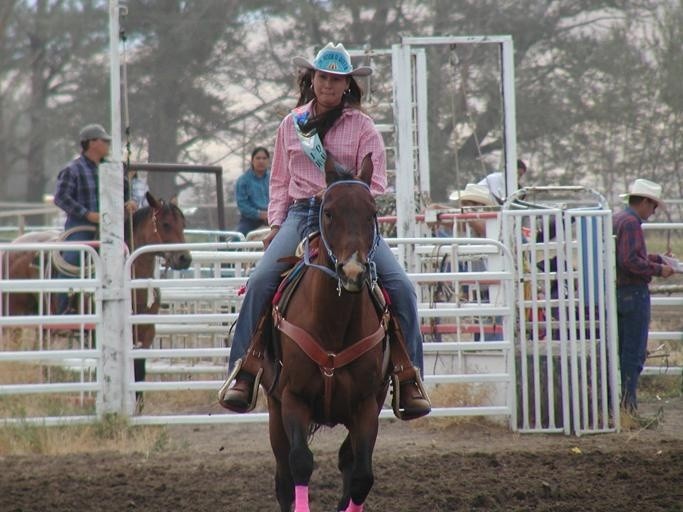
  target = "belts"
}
[293,196,321,205]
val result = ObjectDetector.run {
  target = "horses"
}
[0,191,193,416]
[263,149,392,512]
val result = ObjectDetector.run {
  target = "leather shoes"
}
[223,379,253,409]
[390,384,430,417]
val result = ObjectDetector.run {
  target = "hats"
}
[618,178,666,212]
[292,41,372,76]
[79,124,111,142]
[450,183,495,207]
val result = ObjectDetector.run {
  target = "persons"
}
[477,160,527,207]
[421,182,553,341]
[222,44,433,416]
[230,147,271,245]
[612,179,678,426]
[53,125,151,314]
